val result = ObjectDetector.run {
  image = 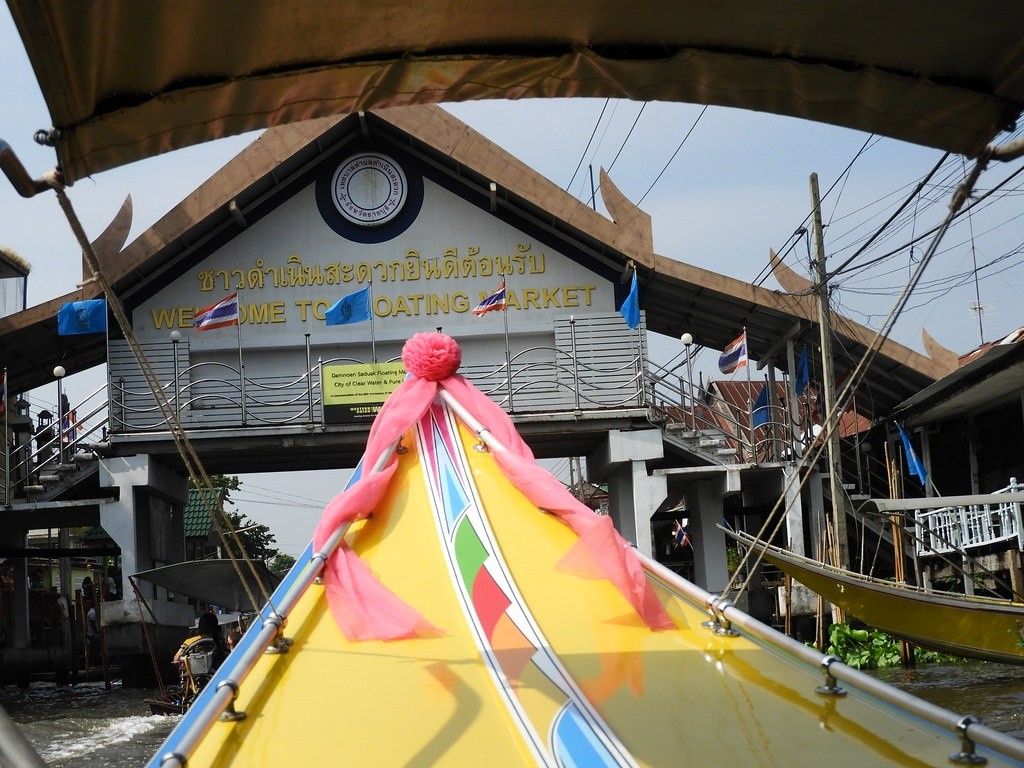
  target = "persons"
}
[107,570,119,600]
[173,613,229,703]
[86,601,100,663]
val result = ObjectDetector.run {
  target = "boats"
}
[128,559,276,716]
[715,489,1024,664]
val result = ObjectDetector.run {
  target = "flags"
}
[324,286,372,326]
[194,292,239,332]
[57,299,106,336]
[619,271,641,333]
[471,282,506,318]
[718,332,748,376]
[674,521,689,547]
[57,411,83,443]
[0,373,7,414]
[896,424,927,485]
[752,383,770,428]
[796,347,810,397]
